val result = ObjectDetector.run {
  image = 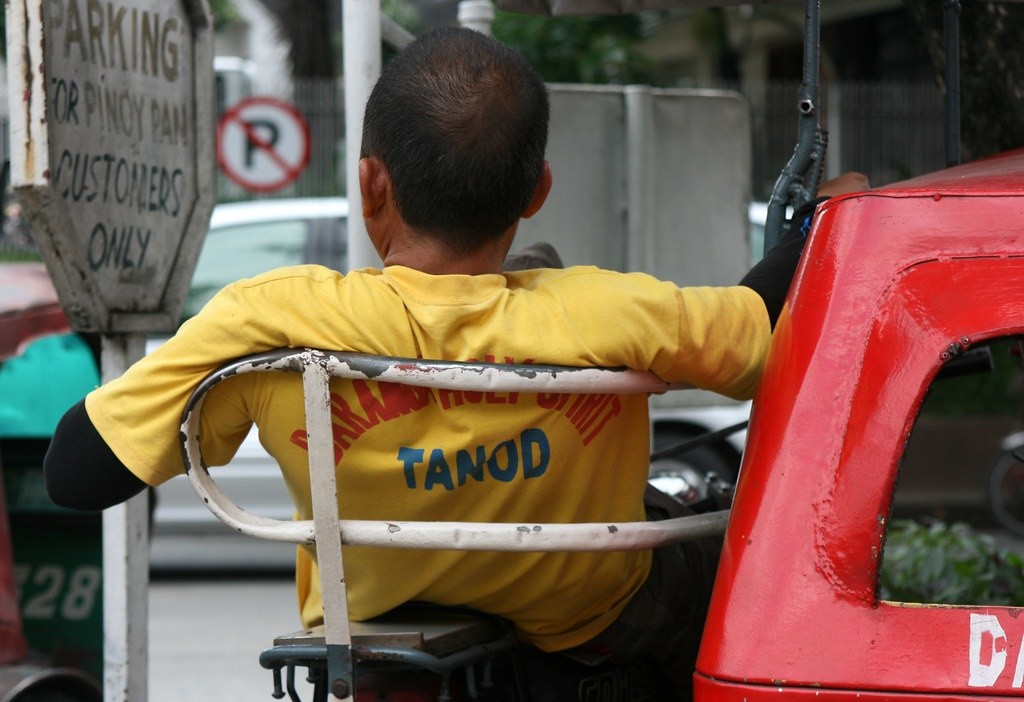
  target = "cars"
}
[141,194,822,586]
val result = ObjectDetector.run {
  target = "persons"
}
[42,20,873,702]
